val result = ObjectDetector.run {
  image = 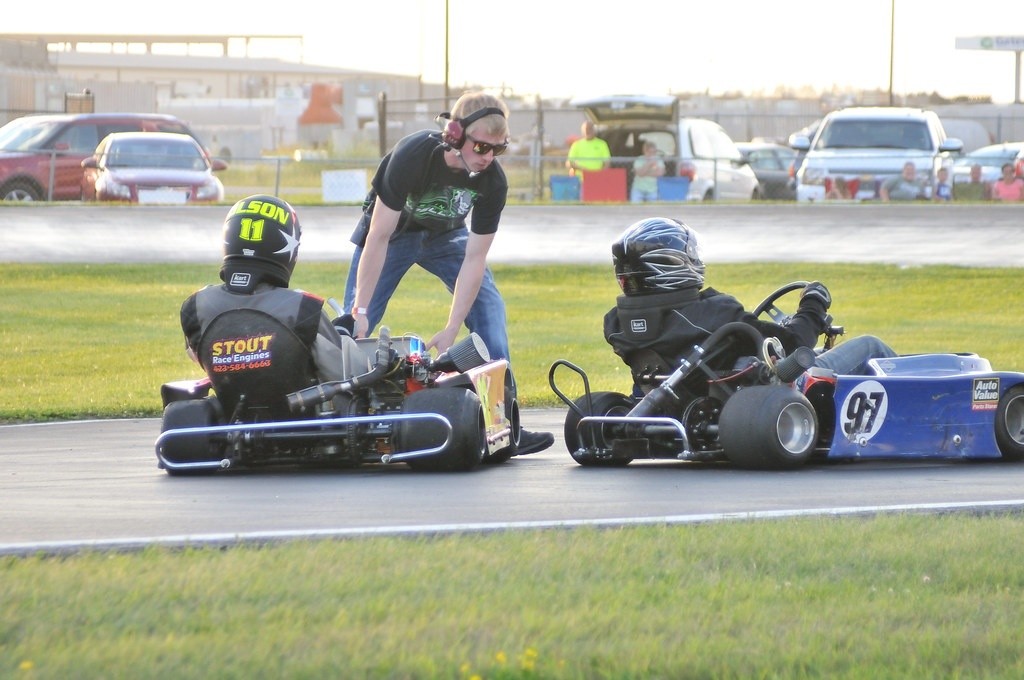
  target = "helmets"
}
[611,216,705,295]
[218,194,301,293]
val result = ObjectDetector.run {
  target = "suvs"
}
[795,107,962,203]
[0,113,212,203]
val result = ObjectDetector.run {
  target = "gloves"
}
[799,281,832,313]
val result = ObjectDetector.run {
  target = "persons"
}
[566,118,613,182]
[341,93,558,465]
[881,158,1024,206]
[603,216,899,382]
[631,138,668,204]
[181,193,359,419]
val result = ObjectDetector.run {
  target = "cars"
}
[734,139,800,196]
[576,91,760,203]
[80,132,229,203]
[954,140,1024,184]
[787,118,822,189]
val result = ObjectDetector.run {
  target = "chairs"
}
[115,145,137,165]
[197,309,314,413]
[629,350,690,414]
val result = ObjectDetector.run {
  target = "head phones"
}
[441,106,505,152]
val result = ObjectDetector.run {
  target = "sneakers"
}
[512,428,555,455]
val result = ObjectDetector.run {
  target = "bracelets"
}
[351,306,368,315]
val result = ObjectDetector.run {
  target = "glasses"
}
[466,135,508,156]
